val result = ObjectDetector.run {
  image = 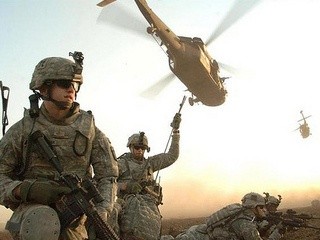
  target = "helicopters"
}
[296,110,313,138]
[96,0,242,108]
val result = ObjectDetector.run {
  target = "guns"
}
[24,131,121,240]
[256,212,320,234]
[270,209,320,221]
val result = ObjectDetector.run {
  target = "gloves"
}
[19,180,71,204]
[87,211,107,240]
[277,222,287,235]
[170,113,181,129]
[126,183,141,194]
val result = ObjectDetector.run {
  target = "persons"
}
[262,192,282,213]
[158,192,267,240]
[0,56,119,240]
[116,114,181,198]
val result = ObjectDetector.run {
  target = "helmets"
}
[264,195,279,205]
[127,133,148,149]
[30,57,83,90]
[242,192,265,207]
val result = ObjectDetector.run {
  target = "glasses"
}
[46,80,79,91]
[129,146,146,150]
[257,205,264,209]
[269,204,278,208]
